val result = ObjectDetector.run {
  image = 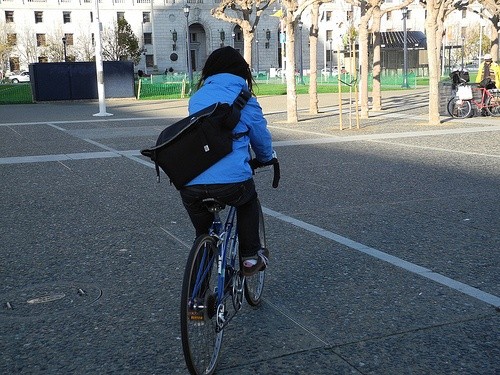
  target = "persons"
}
[332,67,338,77]
[169,67,173,72]
[475,54,500,117]
[138,70,148,77]
[449,66,469,115]
[179,46,278,313]
[341,67,347,73]
[165,68,168,75]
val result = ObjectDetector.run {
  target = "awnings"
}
[380,31,427,48]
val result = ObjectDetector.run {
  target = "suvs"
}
[9,71,30,84]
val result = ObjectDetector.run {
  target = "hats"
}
[483,53,492,60]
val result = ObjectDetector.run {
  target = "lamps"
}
[218,27,225,43]
[266,29,271,42]
[170,28,177,41]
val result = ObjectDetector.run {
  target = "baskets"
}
[437,77,453,96]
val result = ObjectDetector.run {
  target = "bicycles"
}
[446,73,500,119]
[180,156,281,375]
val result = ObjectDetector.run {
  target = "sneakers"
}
[241,247,270,276]
[186,296,206,316]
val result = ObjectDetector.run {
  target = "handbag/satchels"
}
[457,85,473,100]
[141,86,252,189]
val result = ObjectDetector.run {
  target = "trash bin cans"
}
[438,79,455,117]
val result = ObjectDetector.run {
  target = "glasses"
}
[486,60,491,62]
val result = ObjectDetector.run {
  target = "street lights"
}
[181,4,195,97]
[400,6,410,89]
[62,37,68,62]
[297,20,305,85]
